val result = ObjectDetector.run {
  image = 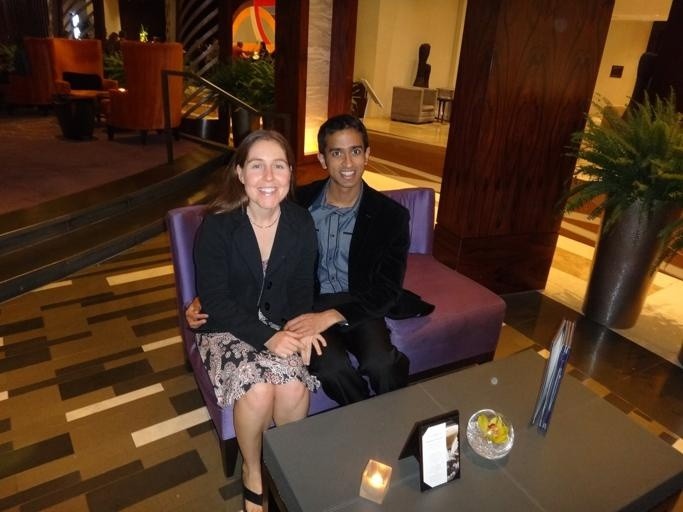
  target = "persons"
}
[188,115,435,406]
[185,130,327,512]
[233,40,269,61]
[108,30,128,54]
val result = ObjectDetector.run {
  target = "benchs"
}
[167,187,507,474]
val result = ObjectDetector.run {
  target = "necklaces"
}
[247,205,280,229]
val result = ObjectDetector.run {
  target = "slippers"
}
[242,463,263,512]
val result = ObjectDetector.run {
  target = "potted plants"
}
[205,58,275,147]
[556,79,682,330]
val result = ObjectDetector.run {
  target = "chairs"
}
[22,34,184,146]
